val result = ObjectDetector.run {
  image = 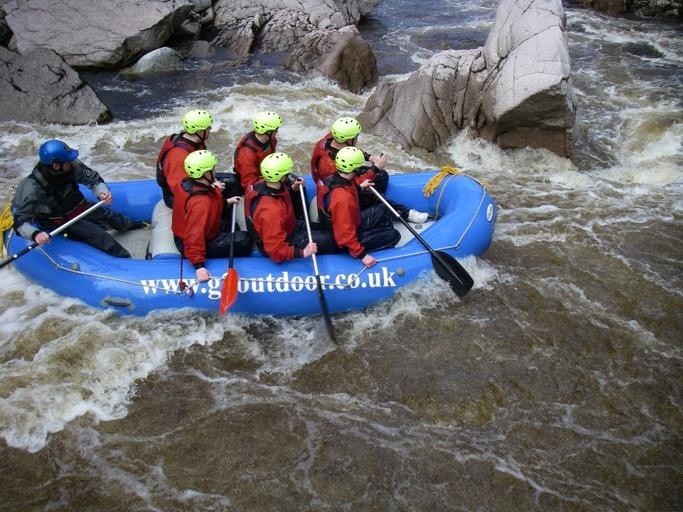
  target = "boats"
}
[0,165,498,319]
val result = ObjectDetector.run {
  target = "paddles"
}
[219,202,239,317]
[298,183,341,347]
[369,185,474,298]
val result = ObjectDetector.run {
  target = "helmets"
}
[259,152,294,184]
[334,145,366,174]
[39,140,79,168]
[330,117,362,144]
[182,109,214,135]
[252,110,283,136]
[183,149,219,180]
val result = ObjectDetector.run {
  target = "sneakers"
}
[406,208,428,223]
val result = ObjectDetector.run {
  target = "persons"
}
[319,146,401,268]
[310,117,389,207]
[244,152,337,265]
[234,111,283,192]
[12,140,153,259]
[170,151,252,282]
[156,109,244,209]
